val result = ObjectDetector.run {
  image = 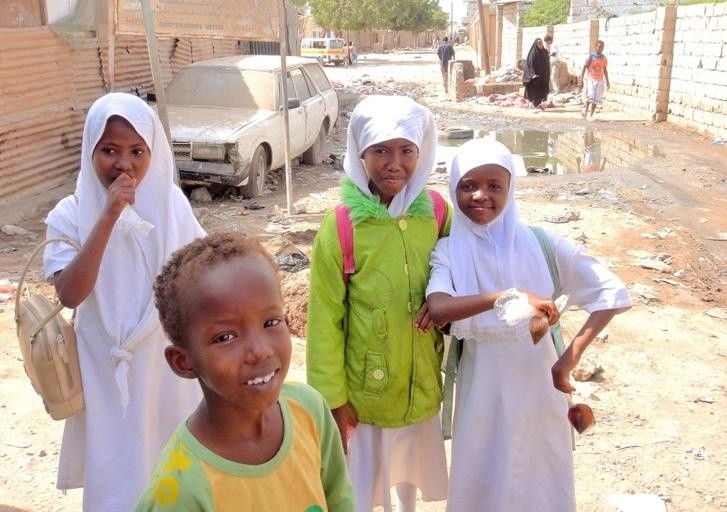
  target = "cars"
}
[147,54,341,198]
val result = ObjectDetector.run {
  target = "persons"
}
[341,41,356,67]
[543,36,556,93]
[575,126,607,174]
[433,35,454,49]
[579,40,610,121]
[437,36,455,93]
[43,93,209,512]
[520,130,549,171]
[133,230,357,512]
[523,37,551,111]
[306,94,454,512]
[425,137,633,512]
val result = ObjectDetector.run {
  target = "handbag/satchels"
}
[15,236,86,421]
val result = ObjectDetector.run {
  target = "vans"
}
[299,38,346,66]
